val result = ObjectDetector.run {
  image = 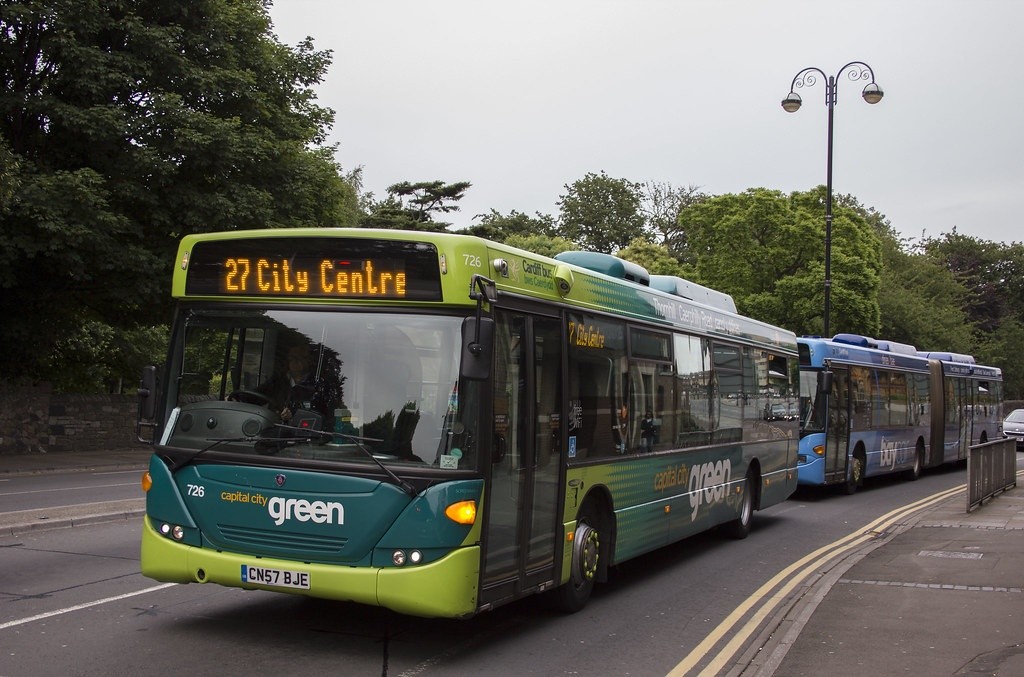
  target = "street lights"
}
[777,62,883,339]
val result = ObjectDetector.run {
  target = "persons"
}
[638,407,656,454]
[223,343,324,425]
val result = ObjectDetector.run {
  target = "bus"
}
[918,351,1005,468]
[137,226,800,624]
[795,333,934,494]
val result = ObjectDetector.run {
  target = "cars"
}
[1001,409,1024,449]
